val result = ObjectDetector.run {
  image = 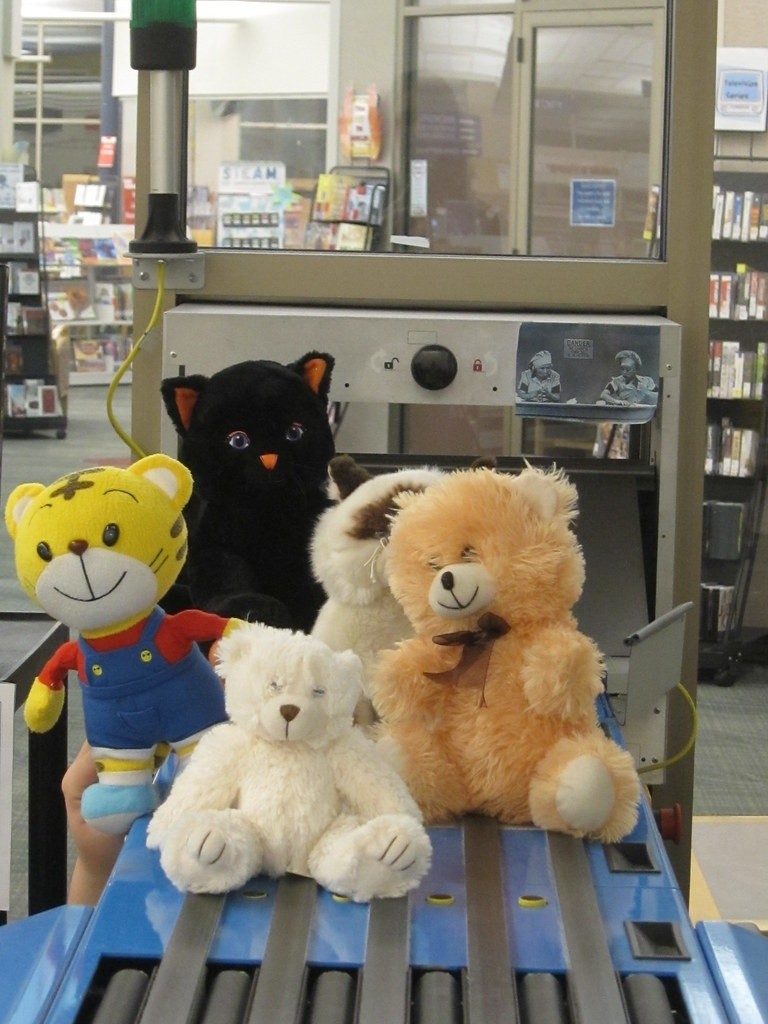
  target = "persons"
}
[61,742,126,910]
[600,349,658,407]
[518,352,562,402]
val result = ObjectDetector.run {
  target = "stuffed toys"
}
[158,352,341,636]
[373,464,642,841]
[310,454,458,775]
[145,620,435,901]
[4,453,234,836]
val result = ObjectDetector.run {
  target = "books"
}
[709,263,768,322]
[705,500,753,558]
[306,172,385,251]
[700,579,736,631]
[707,342,768,401]
[711,184,767,245]
[39,184,135,373]
[703,416,760,478]
[0,164,67,417]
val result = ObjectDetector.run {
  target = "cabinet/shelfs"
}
[0,159,289,440]
[698,172,768,690]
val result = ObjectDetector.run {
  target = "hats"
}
[529,350,552,369]
[615,350,643,371]
